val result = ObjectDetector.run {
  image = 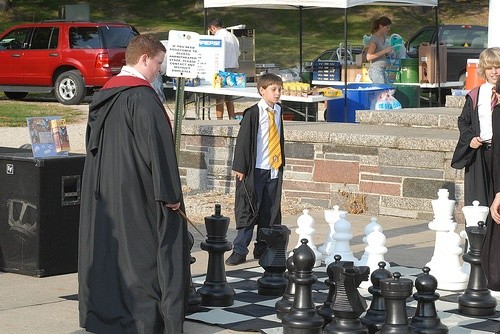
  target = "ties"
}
[491,87,500,112]
[267,109,282,170]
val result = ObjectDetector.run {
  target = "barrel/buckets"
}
[396,85,420,108]
[400,58,421,83]
[466,58,487,90]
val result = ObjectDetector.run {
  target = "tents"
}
[202,0,440,123]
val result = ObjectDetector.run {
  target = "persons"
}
[78,34,190,334]
[208,18,241,120]
[489,192,500,224]
[366,16,393,84]
[451,47,500,292]
[225,72,286,266]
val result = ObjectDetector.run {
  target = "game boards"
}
[60,257,500,334]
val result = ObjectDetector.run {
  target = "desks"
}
[312,81,462,122]
[175,86,344,121]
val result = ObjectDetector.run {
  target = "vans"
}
[0,18,145,105]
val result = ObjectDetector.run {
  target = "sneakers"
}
[253,247,262,259]
[226,252,246,266]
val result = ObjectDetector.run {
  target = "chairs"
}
[470,37,486,49]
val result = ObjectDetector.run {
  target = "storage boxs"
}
[313,61,340,81]
[420,44,447,83]
[465,59,486,90]
[328,84,396,123]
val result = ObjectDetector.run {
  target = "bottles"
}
[395,68,400,83]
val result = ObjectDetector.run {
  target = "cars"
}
[302,42,368,72]
[403,23,488,58]
